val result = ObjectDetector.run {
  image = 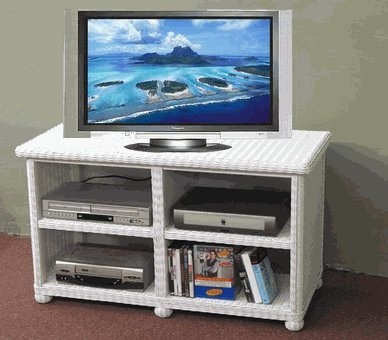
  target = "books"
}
[194,282,241,300]
[192,244,237,288]
[166,240,194,298]
[233,246,280,304]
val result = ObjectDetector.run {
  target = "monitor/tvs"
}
[61,6,294,153]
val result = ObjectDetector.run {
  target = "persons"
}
[201,249,220,276]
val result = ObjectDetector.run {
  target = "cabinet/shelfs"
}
[15,131,332,330]
